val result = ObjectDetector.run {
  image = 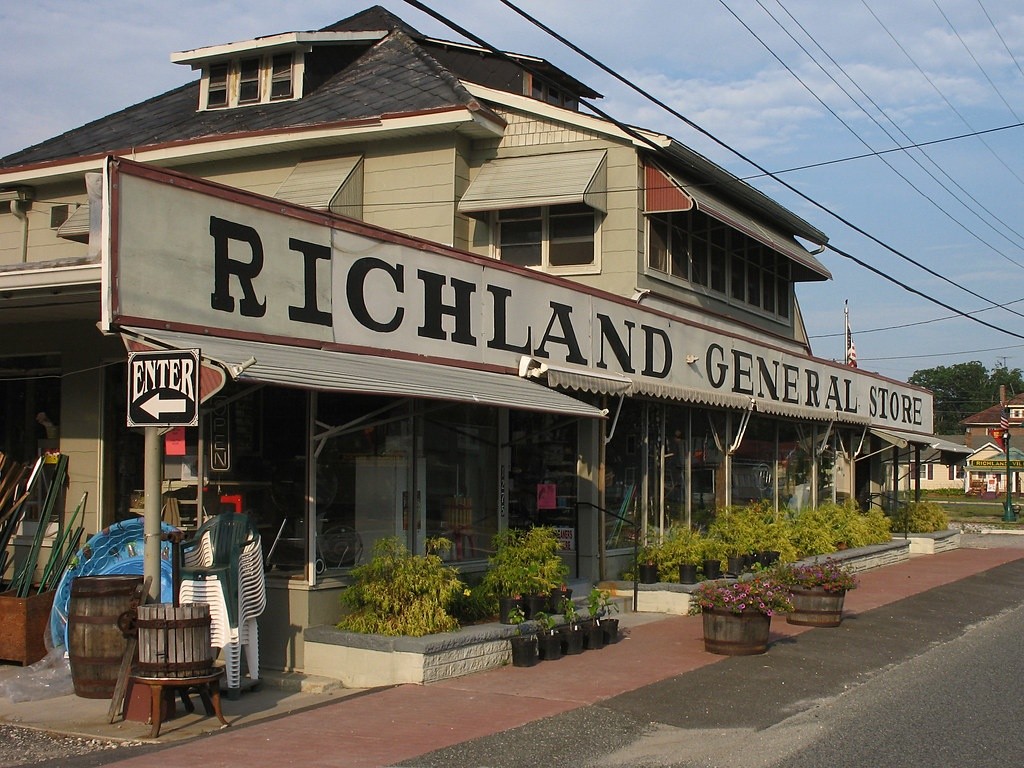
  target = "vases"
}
[703,606,770,656]
[781,586,845,628]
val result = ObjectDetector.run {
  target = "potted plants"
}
[472,525,619,667]
[637,500,781,584]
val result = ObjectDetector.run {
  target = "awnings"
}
[122,325,607,605]
[869,427,972,454]
[644,158,833,282]
[519,354,870,456]
[56,152,363,246]
[457,149,607,222]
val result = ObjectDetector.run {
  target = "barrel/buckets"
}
[137,604,212,678]
[68,575,146,699]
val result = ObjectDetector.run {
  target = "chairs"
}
[173,511,268,691]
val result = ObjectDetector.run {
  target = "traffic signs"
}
[127,347,198,427]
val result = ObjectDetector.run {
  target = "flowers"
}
[686,573,789,617]
[774,560,858,592]
